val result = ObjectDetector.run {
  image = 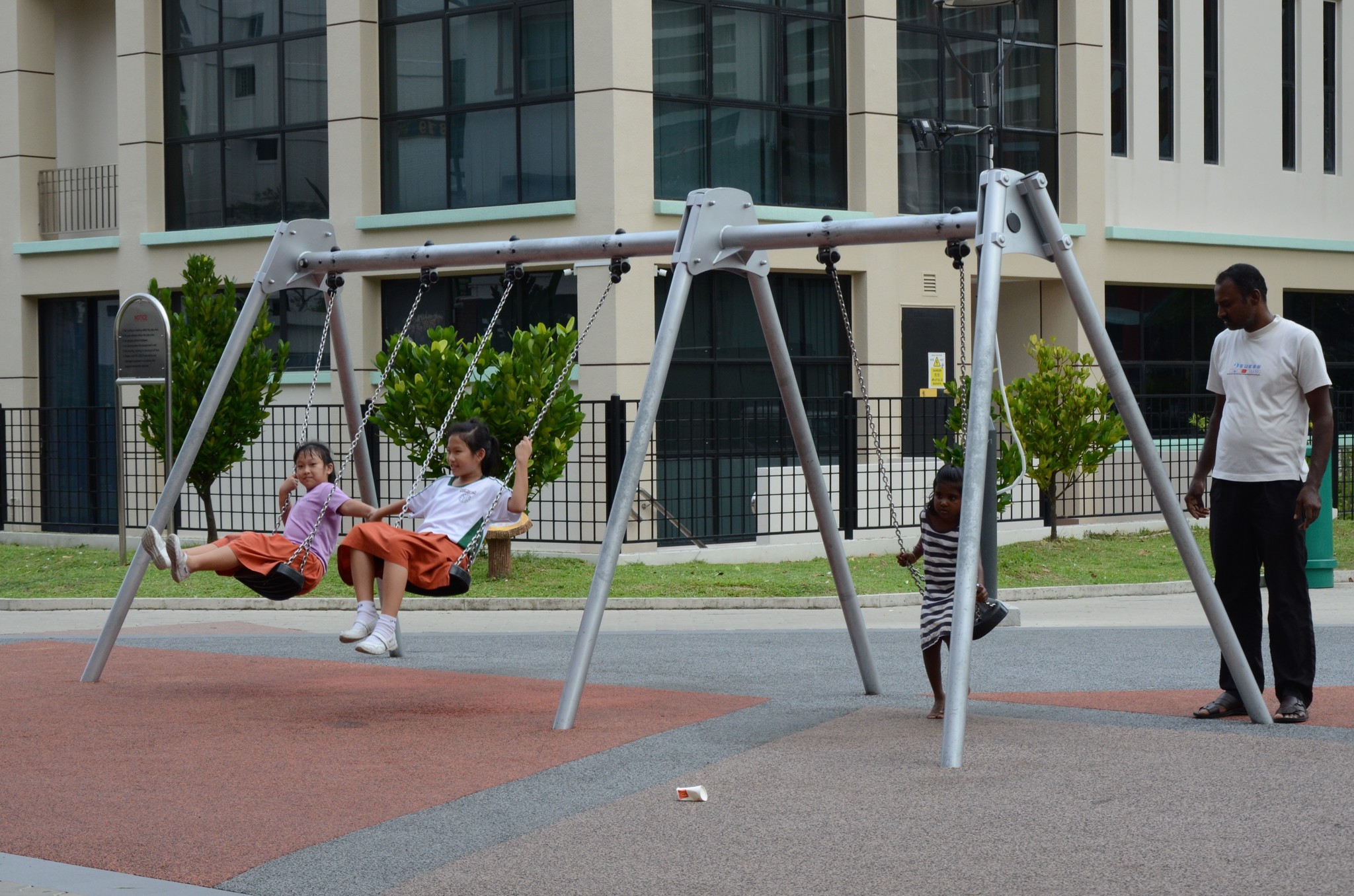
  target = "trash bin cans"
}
[1304,446,1337,588]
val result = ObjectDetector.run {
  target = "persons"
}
[897,463,990,719]
[1185,266,1336,724]
[338,418,533,654]
[142,441,381,596]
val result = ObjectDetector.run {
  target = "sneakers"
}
[355,620,398,655]
[339,607,380,643]
[165,533,191,583]
[141,525,171,571]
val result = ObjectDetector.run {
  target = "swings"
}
[231,266,439,602]
[370,254,631,598]
[816,238,1009,641]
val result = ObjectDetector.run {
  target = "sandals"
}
[1193,690,1249,718]
[1273,696,1309,721]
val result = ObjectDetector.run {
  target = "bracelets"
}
[977,583,984,587]
[911,552,917,562]
